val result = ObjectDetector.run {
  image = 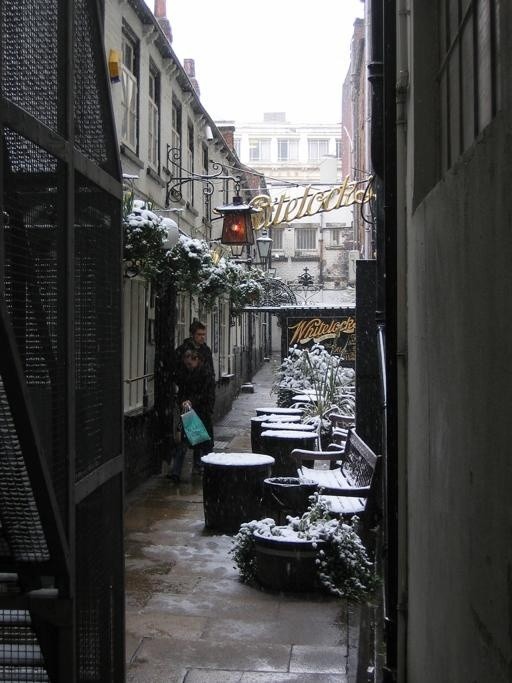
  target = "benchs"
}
[287,428,382,548]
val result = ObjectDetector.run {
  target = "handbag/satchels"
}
[181,410,211,446]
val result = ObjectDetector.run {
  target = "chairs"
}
[328,413,357,470]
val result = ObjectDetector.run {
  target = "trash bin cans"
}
[264,477,318,526]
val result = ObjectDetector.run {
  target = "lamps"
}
[235,228,271,267]
[211,236,243,259]
[165,145,255,257]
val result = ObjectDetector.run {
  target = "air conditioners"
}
[348,250,359,285]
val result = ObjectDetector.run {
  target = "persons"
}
[166,346,217,483]
[165,319,216,468]
